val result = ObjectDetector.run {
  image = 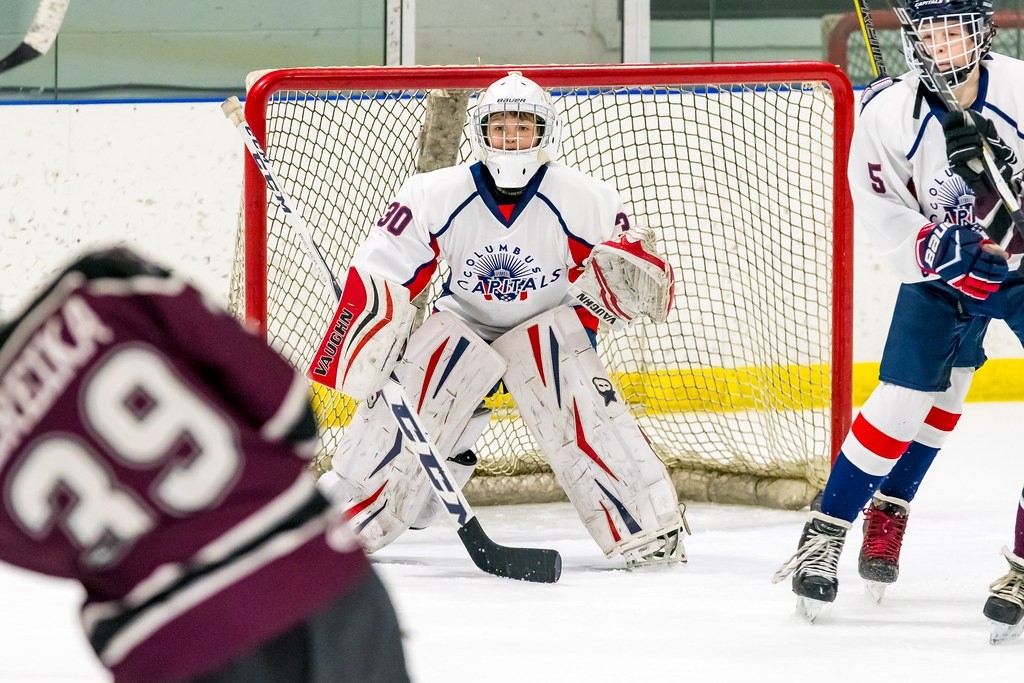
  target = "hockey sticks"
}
[853,0,1024,240]
[219,95,566,586]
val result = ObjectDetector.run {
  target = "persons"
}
[0,244,414,683]
[306,72,692,568]
[772,0,1024,645]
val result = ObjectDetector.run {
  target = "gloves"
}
[943,109,1019,210]
[915,221,1011,300]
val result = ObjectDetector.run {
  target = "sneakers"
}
[771,489,853,624]
[983,546,1024,645]
[858,489,911,605]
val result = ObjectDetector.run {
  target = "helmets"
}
[901,0,998,91]
[469,75,558,188]
